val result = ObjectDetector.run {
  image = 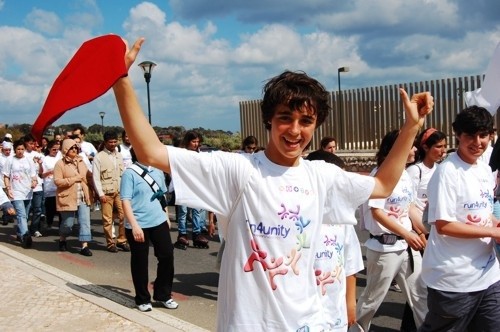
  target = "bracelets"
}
[122,74,128,78]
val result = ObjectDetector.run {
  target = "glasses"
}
[69,147,78,150]
[247,146,255,151]
[53,149,58,151]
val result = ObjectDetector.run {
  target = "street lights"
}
[338,67,351,150]
[137,60,158,124]
[98,112,106,128]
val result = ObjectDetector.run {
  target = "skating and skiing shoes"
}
[192,234,209,248]
[174,234,189,250]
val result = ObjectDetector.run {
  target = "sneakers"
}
[136,302,152,312]
[160,299,178,308]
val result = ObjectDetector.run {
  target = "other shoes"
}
[34,231,42,237]
[80,247,92,256]
[16,235,33,247]
[59,238,67,251]
[106,246,118,252]
[2,218,14,225]
[389,284,402,293]
[112,233,116,239]
[117,243,131,252]
[202,229,208,236]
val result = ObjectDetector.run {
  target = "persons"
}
[0,105,500,332]
[103,35,435,332]
